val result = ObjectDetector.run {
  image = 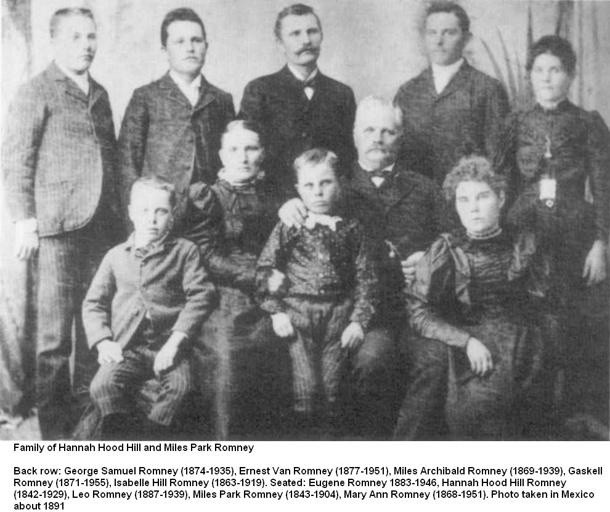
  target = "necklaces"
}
[469,226,500,237]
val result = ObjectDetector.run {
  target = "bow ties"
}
[367,169,392,180]
[295,78,319,89]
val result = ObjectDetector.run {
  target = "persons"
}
[183,118,288,440]
[492,34,610,307]
[394,3,511,187]
[404,154,574,441]
[254,147,379,417]
[4,7,119,441]
[237,4,356,199]
[81,177,214,441]
[112,7,235,223]
[277,95,447,441]
